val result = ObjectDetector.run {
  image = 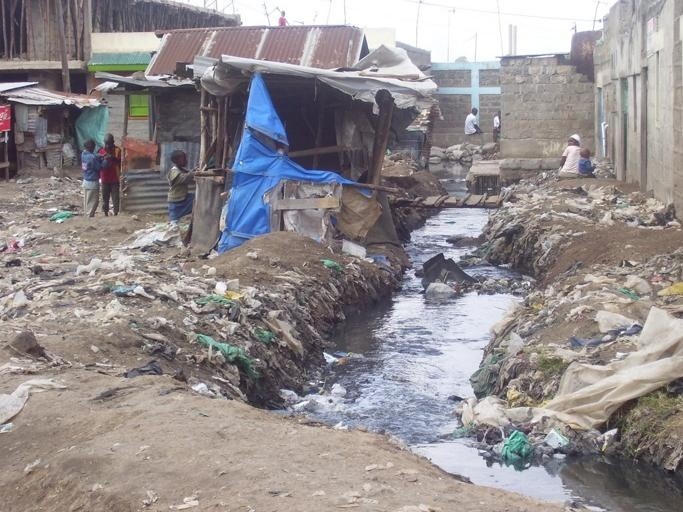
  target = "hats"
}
[570,134,580,142]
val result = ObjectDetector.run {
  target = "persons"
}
[167,149,200,220]
[491,111,501,144]
[80,138,108,218]
[96,133,122,218]
[556,133,584,179]
[277,10,290,26]
[578,147,597,178]
[464,108,482,135]
